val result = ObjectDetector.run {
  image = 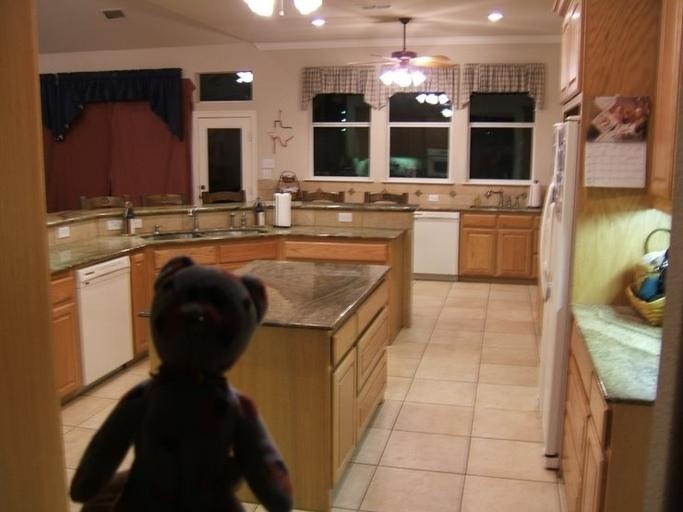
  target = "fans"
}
[349,18,452,67]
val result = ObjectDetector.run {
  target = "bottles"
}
[254,198,264,225]
[125,206,135,234]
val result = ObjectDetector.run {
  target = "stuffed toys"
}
[71,258,293,511]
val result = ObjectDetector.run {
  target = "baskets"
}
[624,228,672,327]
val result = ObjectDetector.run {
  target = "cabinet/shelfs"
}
[540,0,661,470]
[47,273,82,407]
[459,209,539,282]
[122,251,156,358]
[279,238,400,346]
[558,303,664,510]
[224,257,395,512]
[72,256,136,389]
[151,236,279,290]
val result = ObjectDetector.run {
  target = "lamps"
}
[244,0,273,17]
[377,64,428,91]
[293,0,322,15]
[415,92,450,109]
